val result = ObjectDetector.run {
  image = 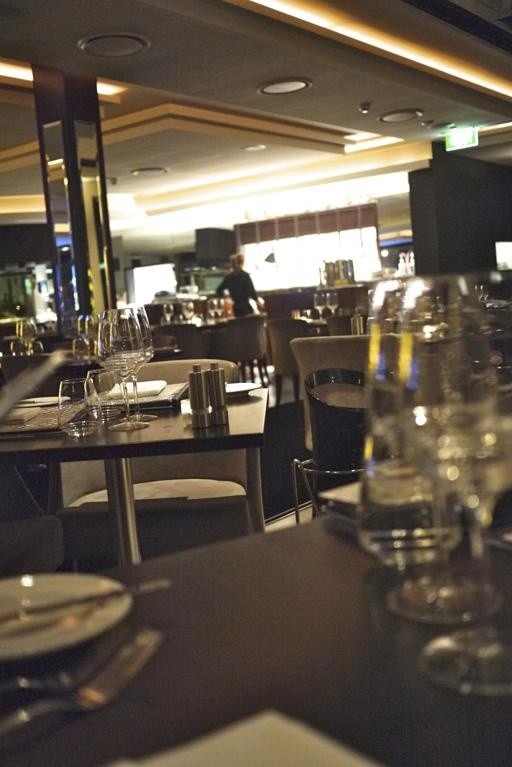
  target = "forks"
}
[2,628,165,736]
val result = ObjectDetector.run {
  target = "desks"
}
[0,514,512,767]
[0,388,268,566]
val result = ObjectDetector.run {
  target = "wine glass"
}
[112,305,158,423]
[95,308,150,431]
[289,270,511,698]
[16,272,236,364]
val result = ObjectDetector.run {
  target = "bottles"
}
[206,362,227,410]
[186,364,210,413]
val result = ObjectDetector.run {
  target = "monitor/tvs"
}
[408,154,512,275]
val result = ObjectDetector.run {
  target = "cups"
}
[57,379,104,437]
[85,369,124,422]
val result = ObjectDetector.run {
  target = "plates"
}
[225,382,262,398]
[0,572,134,663]
[15,396,71,409]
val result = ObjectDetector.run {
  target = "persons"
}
[216,252,264,316]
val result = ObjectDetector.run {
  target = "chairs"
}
[0,290,512,524]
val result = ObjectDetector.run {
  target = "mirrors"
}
[41,122,81,315]
[73,118,113,322]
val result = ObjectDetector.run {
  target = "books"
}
[88,381,189,409]
[0,402,91,435]
[5,405,37,424]
